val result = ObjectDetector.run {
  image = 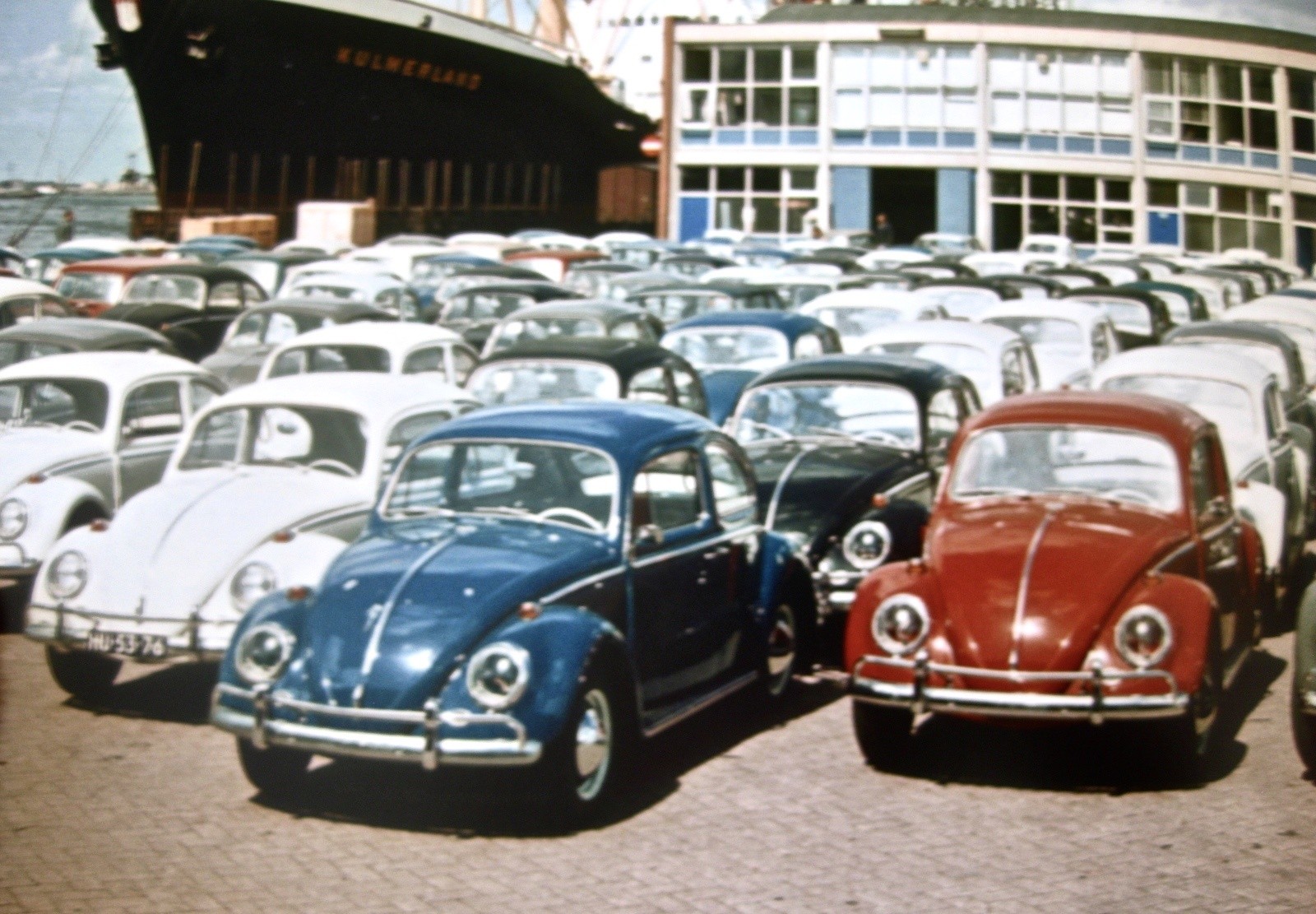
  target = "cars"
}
[17,370,491,701]
[206,396,821,838]
[1,349,235,637]
[3,227,1316,432]
[718,351,987,613]
[844,390,1269,791]
[1046,343,1312,614]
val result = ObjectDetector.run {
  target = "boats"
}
[86,0,664,246]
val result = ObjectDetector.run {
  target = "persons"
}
[872,214,893,248]
[56,209,72,243]
